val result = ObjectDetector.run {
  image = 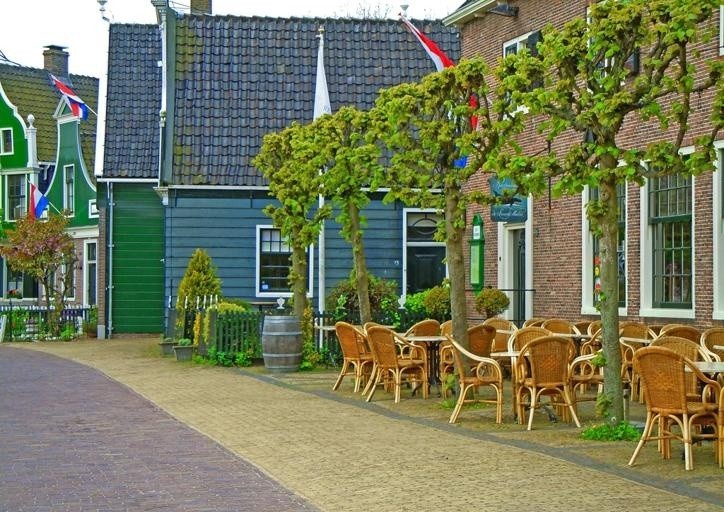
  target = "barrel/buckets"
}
[260,313,304,375]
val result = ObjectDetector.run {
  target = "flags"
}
[29,183,49,224]
[50,74,88,121]
[402,16,479,170]
[311,34,333,124]
